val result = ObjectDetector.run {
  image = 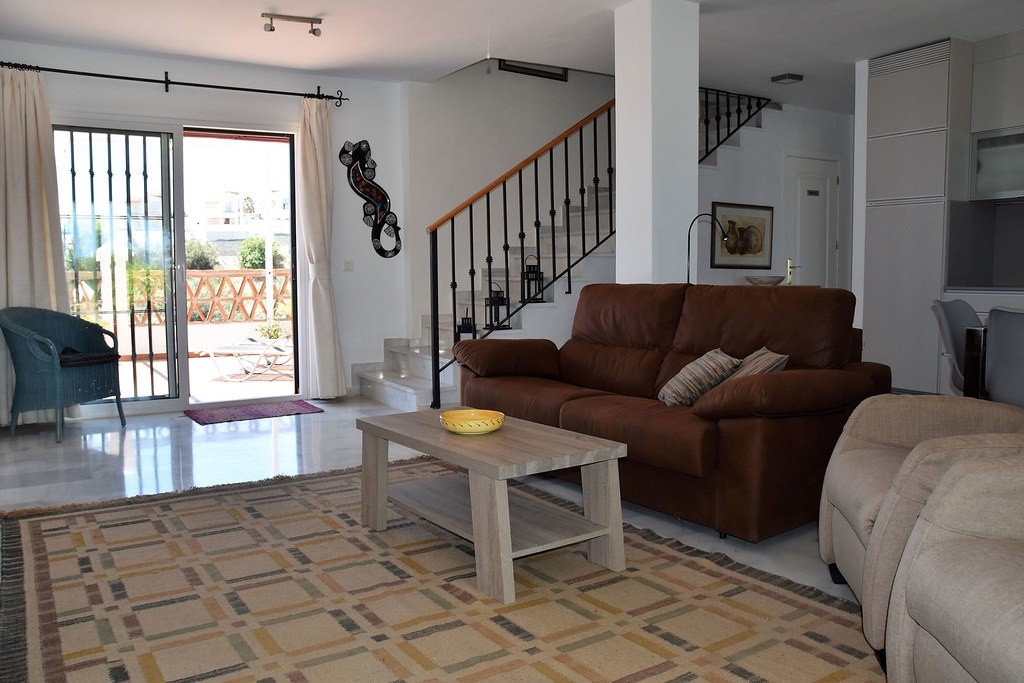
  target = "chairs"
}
[199,331,292,381]
[0,305,127,442]
[932,298,1024,408]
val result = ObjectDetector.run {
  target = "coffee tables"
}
[356,404,626,604]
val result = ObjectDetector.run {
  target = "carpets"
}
[183,399,324,426]
[1,454,889,683]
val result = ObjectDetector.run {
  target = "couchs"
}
[818,395,1024,683]
[451,280,891,543]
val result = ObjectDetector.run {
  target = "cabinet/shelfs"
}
[865,31,1024,400]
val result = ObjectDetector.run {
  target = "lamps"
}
[687,212,728,284]
[457,307,474,342]
[482,281,512,331]
[263,16,275,32]
[307,23,322,37]
[519,255,545,307]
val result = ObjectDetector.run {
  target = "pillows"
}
[721,346,790,386]
[657,348,739,406]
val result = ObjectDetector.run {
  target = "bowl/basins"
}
[745,276,785,285]
[440,410,505,434]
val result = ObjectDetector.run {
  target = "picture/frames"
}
[710,202,774,270]
